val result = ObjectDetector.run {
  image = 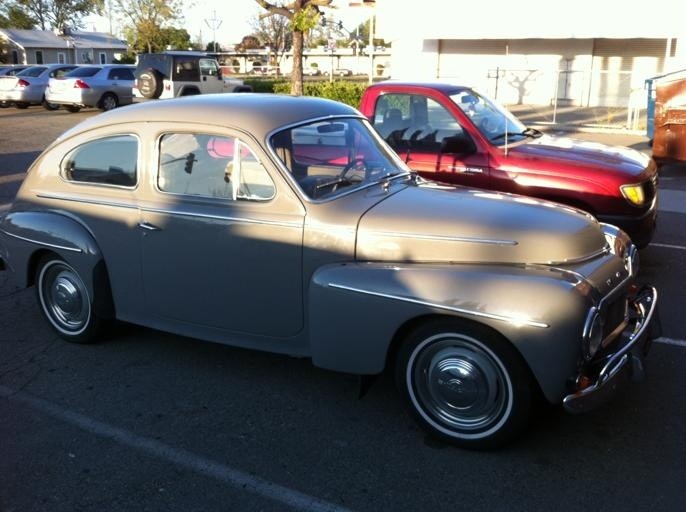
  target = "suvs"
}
[128,49,252,104]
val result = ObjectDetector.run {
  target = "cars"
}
[0,89,673,451]
[0,63,78,111]
[39,60,138,114]
[0,63,32,107]
[243,65,353,76]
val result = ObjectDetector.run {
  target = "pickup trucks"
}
[196,73,663,257]
